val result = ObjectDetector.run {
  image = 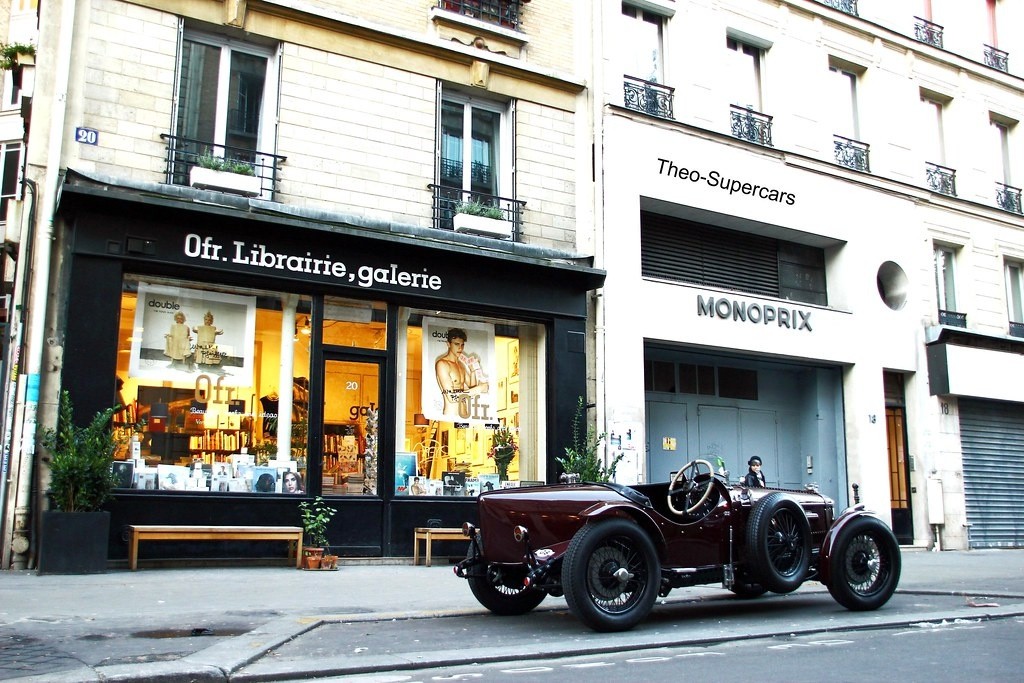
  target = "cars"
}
[447,458,904,630]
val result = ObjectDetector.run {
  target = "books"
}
[322,434,364,495]
[189,413,246,465]
[408,471,545,497]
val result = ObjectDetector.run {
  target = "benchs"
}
[128,524,304,571]
[414,528,480,567]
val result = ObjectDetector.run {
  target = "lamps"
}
[414,414,429,427]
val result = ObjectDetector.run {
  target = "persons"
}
[217,465,228,475]
[411,477,419,496]
[435,328,477,419]
[745,456,765,489]
[283,472,304,494]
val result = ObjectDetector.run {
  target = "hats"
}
[748,456,762,465]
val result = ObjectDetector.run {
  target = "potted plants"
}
[453,195,513,238]
[190,145,261,197]
[0,42,37,70]
[27,390,149,575]
[288,496,338,569]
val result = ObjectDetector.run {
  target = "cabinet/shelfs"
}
[170,427,253,454]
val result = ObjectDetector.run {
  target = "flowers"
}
[487,426,520,481]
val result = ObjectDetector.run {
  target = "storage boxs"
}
[149,416,167,432]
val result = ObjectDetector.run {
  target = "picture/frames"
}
[455,428,466,455]
[509,381,519,409]
[497,377,507,412]
[507,339,519,385]
[507,438,519,473]
[472,427,485,467]
[441,428,450,458]
